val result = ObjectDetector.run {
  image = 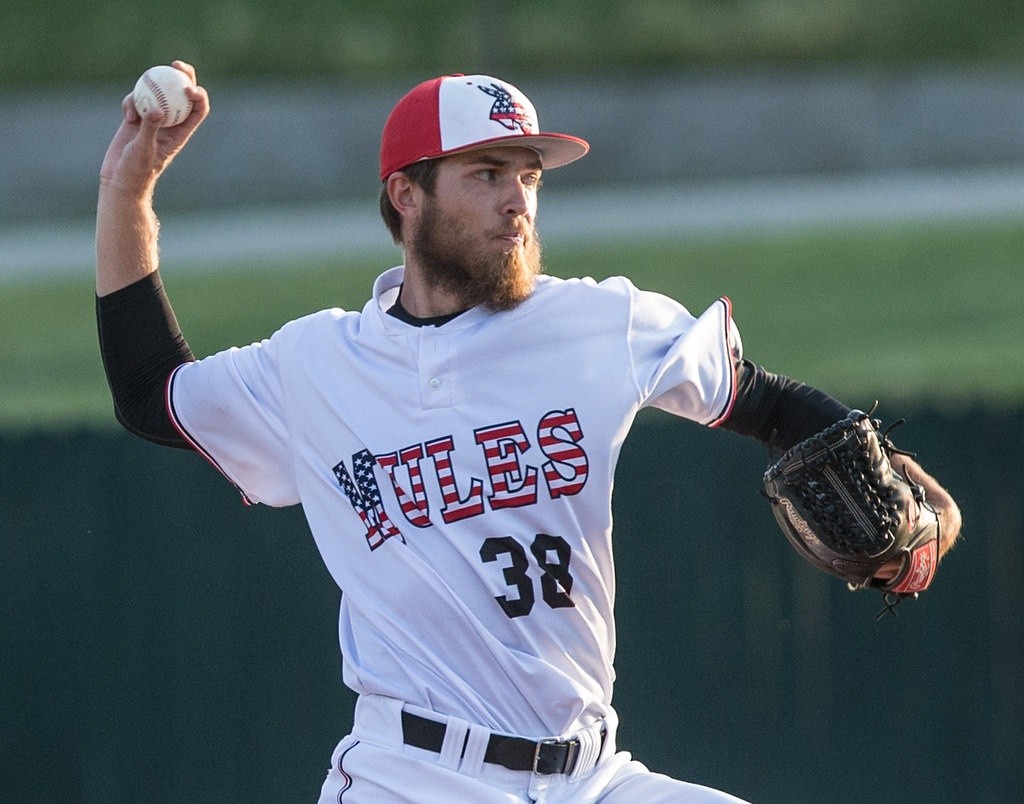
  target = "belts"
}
[401,710,607,775]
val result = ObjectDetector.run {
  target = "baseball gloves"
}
[764,411,942,595]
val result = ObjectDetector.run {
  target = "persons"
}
[93,60,962,803]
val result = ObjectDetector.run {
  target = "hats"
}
[379,72,590,184]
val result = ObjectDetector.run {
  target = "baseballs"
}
[133,65,194,129]
